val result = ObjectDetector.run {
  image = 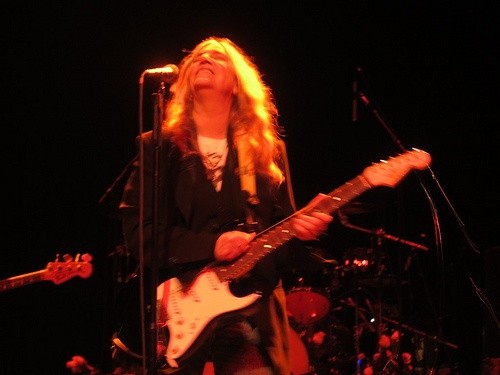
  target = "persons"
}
[116,34,336,375]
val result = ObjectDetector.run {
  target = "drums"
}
[285,286,330,324]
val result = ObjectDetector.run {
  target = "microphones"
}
[145,64,179,79]
[352,65,359,121]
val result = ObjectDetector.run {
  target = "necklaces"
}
[201,143,229,178]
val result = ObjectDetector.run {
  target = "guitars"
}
[0,250,92,291]
[110,148,431,368]
